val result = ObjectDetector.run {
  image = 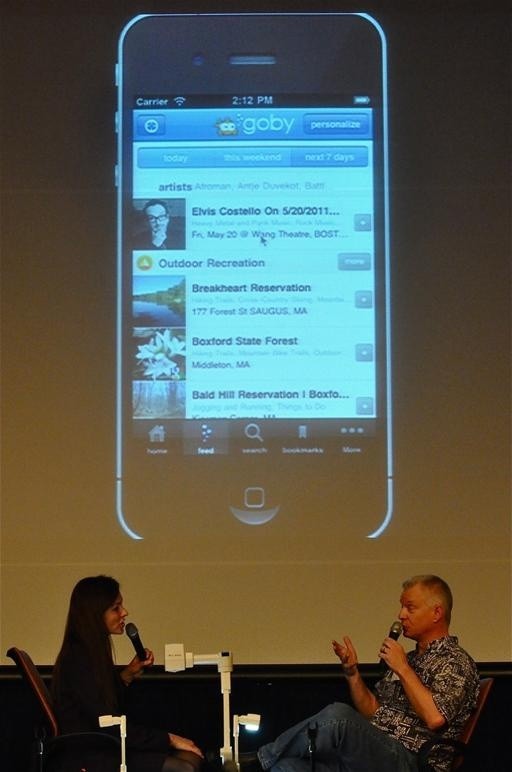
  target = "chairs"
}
[419,675,494,772]
[5,645,128,771]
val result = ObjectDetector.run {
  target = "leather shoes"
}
[240,751,271,772]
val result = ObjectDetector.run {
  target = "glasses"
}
[148,211,168,223]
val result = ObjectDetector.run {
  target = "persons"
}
[50,574,205,772]
[256,574,481,771]
[133,199,179,248]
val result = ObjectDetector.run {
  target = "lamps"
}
[98,714,127,772]
[165,643,262,772]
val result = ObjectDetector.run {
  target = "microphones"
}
[378,621,404,674]
[125,622,149,668]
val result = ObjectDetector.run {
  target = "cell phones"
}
[116,11,395,539]
